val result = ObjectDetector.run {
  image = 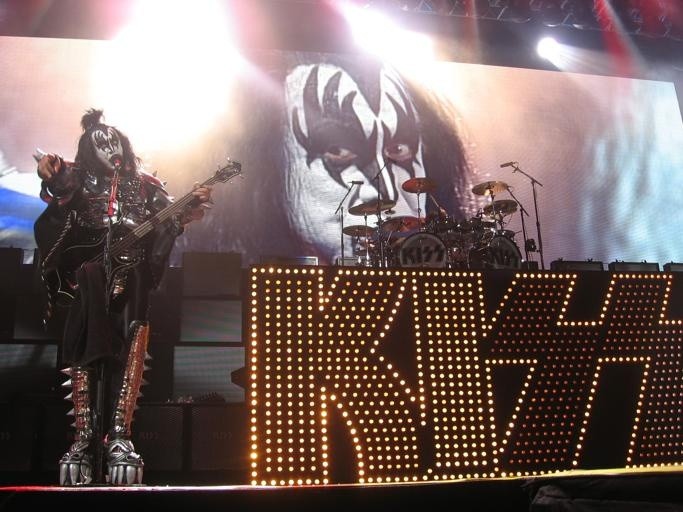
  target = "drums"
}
[380,215,421,253]
[466,236,522,269]
[388,231,448,267]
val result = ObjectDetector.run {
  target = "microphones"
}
[352,181,364,184]
[500,162,514,168]
[386,157,397,164]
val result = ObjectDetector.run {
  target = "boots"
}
[59,366,105,487]
[102,319,152,485]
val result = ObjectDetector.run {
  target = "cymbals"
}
[482,200,518,216]
[342,225,375,236]
[348,200,396,214]
[472,181,508,195]
[402,178,432,193]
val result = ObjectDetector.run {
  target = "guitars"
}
[55,158,246,302]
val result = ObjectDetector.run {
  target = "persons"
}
[31,107,214,487]
[221,52,479,268]
